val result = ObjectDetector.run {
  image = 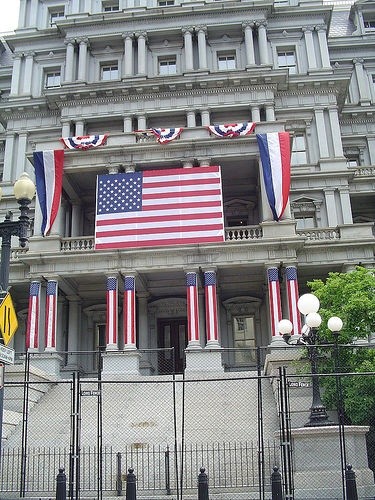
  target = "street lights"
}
[0,171,38,459]
[277,294,345,426]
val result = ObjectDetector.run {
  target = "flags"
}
[33,149,64,237]
[256,131,290,222]
[95,165,225,250]
